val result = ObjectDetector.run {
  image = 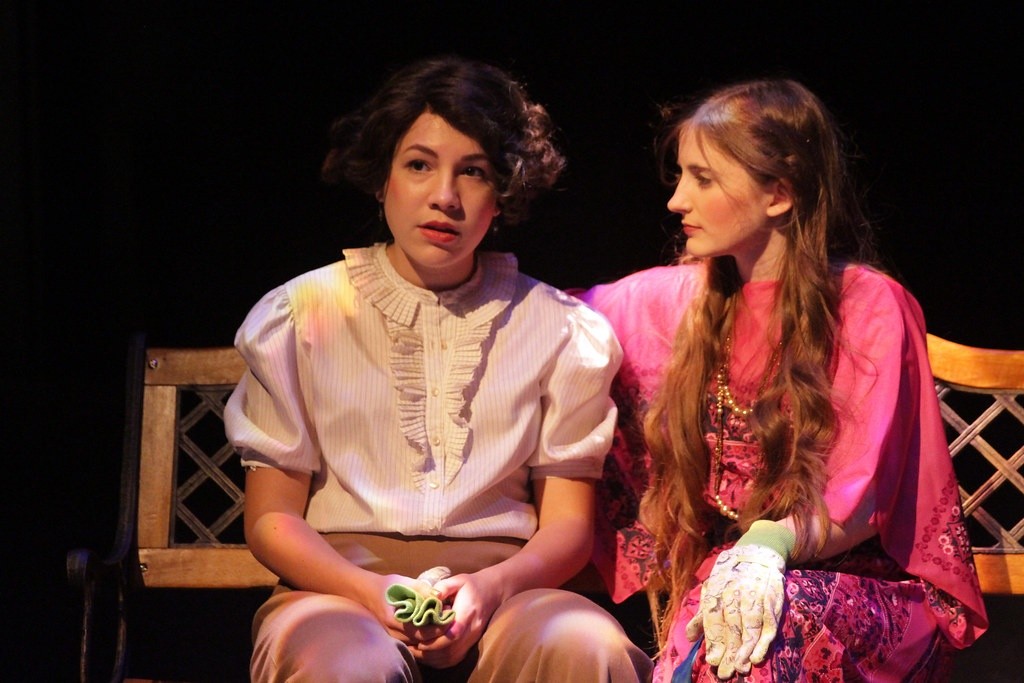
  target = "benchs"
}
[66,332,1023,683]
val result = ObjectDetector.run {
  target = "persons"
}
[556,78,987,683]
[223,59,654,683]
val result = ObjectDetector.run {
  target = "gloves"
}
[686,519,795,678]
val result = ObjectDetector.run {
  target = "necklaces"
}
[713,329,784,521]
